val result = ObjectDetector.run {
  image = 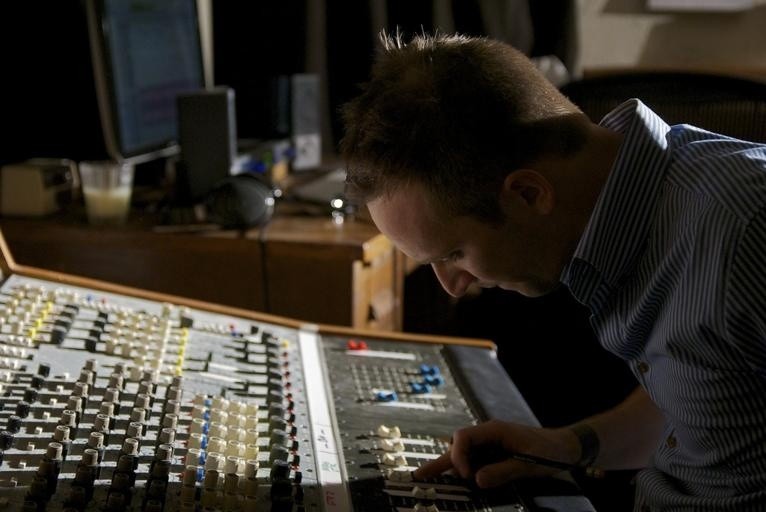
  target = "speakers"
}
[278,73,324,171]
[176,87,237,196]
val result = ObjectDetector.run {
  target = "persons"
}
[343,28,766,512]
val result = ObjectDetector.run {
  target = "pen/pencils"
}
[449,436,577,471]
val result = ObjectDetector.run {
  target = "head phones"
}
[166,172,279,231]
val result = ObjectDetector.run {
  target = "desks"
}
[1,153,418,335]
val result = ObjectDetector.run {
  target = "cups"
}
[78,159,137,229]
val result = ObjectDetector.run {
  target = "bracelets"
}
[567,421,601,477]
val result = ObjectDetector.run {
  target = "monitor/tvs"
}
[85,0,216,167]
